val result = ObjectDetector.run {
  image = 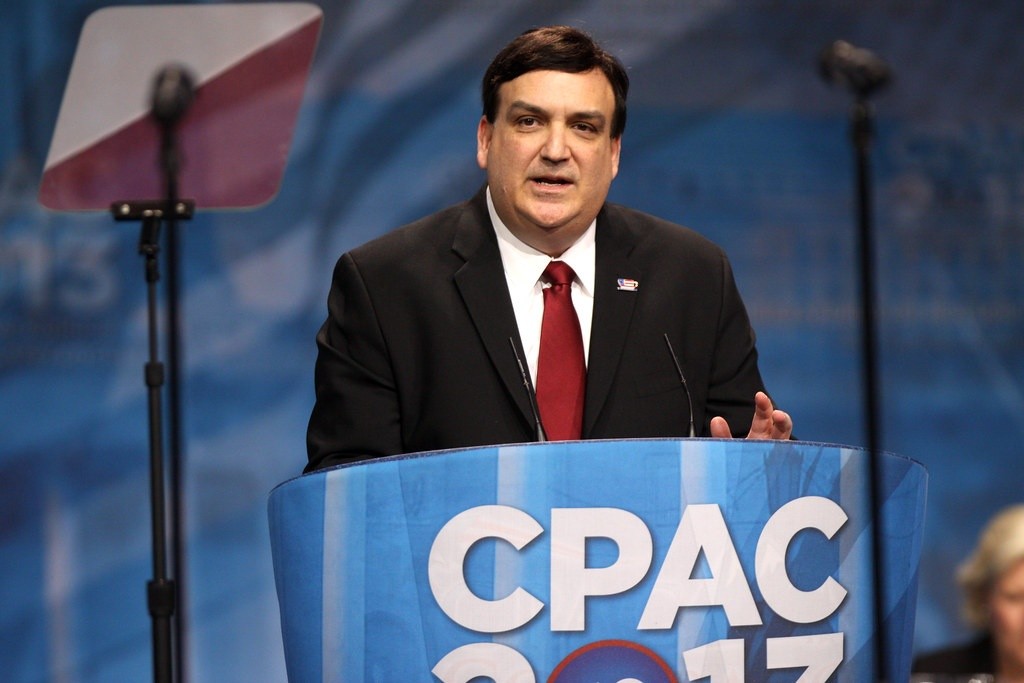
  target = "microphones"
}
[663,334,698,438]
[508,336,548,441]
[819,41,891,93]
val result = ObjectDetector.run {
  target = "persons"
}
[306,25,797,471]
[914,498,1024,681]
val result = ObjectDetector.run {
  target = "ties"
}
[535,261,585,441]
